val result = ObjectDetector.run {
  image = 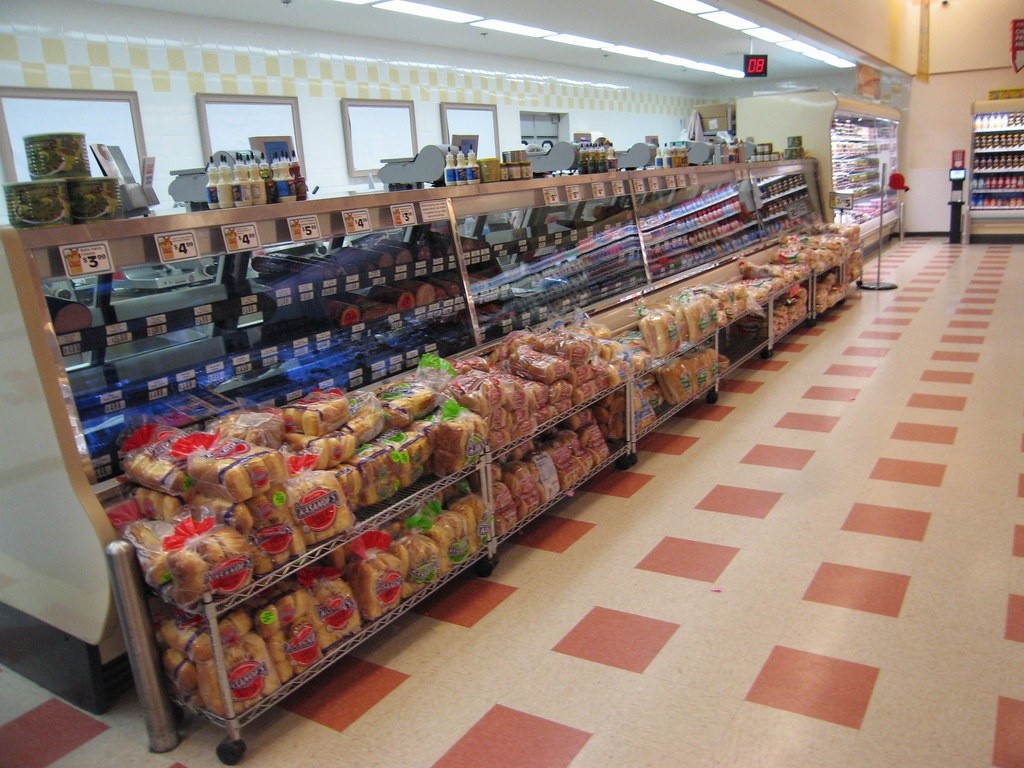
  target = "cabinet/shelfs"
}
[2,159,864,764]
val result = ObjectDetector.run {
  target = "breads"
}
[123,222,866,720]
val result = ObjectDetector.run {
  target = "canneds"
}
[500,161,532,181]
[750,143,780,162]
[783,136,805,160]
